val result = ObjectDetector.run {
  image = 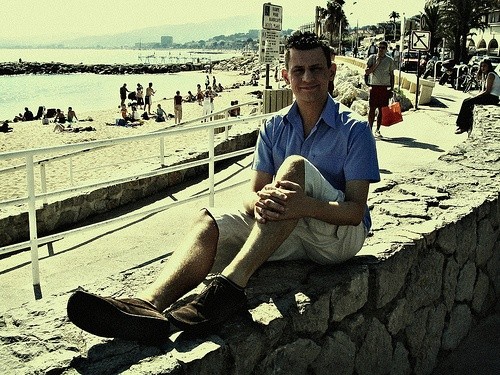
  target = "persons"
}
[228,100,240,117]
[455,57,500,134]
[137,83,144,109]
[144,82,157,113]
[367,41,377,57]
[120,83,131,106]
[365,40,395,138]
[18,105,78,123]
[155,104,168,122]
[387,45,402,58]
[174,90,184,124]
[53,124,97,133]
[120,104,131,126]
[128,104,141,122]
[0,120,14,133]
[67,30,381,337]
[182,75,224,106]
[352,45,358,58]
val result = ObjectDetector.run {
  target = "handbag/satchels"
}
[365,74,370,87]
[381,98,403,126]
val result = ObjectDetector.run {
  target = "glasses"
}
[378,47,386,49]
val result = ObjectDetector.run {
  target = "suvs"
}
[401,49,429,72]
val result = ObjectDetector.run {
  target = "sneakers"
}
[375,131,382,137]
[67,291,169,340]
[162,273,246,330]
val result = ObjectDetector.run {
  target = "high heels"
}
[455,128,468,134]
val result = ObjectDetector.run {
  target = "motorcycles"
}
[422,56,439,78]
[439,58,469,85]
